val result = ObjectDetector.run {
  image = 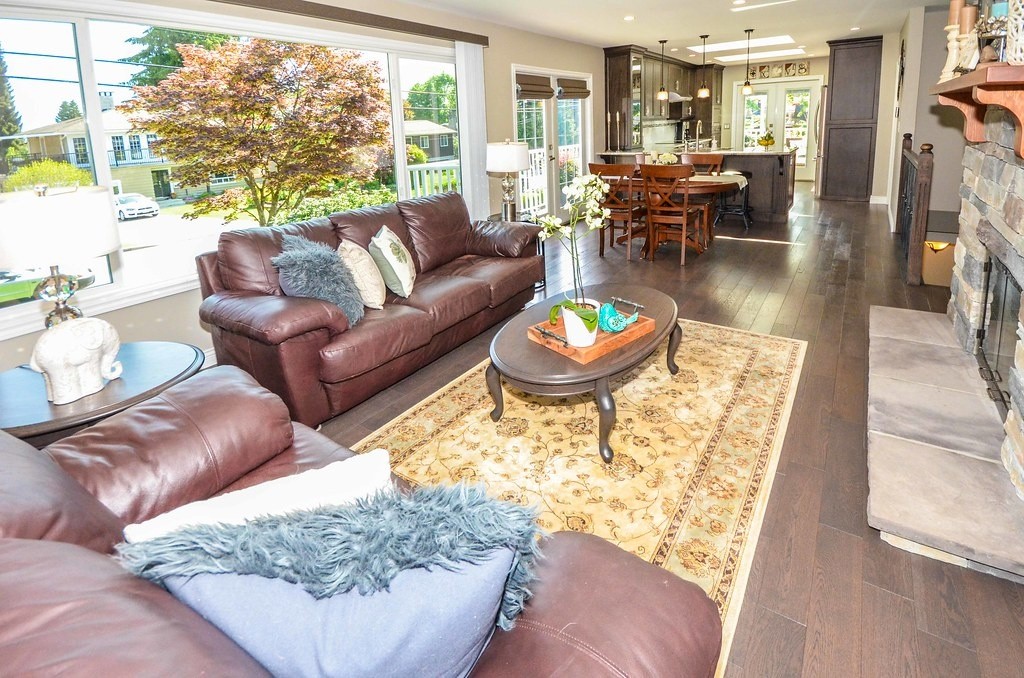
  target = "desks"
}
[601,176,745,253]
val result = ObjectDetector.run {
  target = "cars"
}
[113,193,160,222]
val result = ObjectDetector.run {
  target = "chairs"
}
[588,154,723,266]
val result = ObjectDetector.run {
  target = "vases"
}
[560,296,604,345]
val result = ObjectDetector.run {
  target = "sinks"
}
[670,147,734,152]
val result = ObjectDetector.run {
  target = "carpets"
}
[349,318,808,678]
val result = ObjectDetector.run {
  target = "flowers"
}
[533,172,609,305]
[659,154,678,165]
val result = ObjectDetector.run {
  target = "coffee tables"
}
[486,285,682,463]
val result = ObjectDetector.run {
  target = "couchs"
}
[1,369,723,678]
[194,192,547,430]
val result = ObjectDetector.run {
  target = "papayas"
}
[759,133,775,145]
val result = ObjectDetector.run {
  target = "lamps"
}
[0,189,119,403]
[484,140,532,222]
[656,28,753,100]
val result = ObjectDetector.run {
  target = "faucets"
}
[684,142,690,153]
[695,119,703,152]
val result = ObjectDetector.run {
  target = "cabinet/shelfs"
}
[603,44,724,146]
[713,171,754,230]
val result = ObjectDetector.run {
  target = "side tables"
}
[0,339,204,433]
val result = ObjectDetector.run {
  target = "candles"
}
[607,111,657,164]
[949,0,977,34]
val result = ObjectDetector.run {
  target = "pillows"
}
[108,450,554,678]
[270,225,417,326]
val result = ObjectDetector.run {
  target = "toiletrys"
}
[710,136,717,151]
